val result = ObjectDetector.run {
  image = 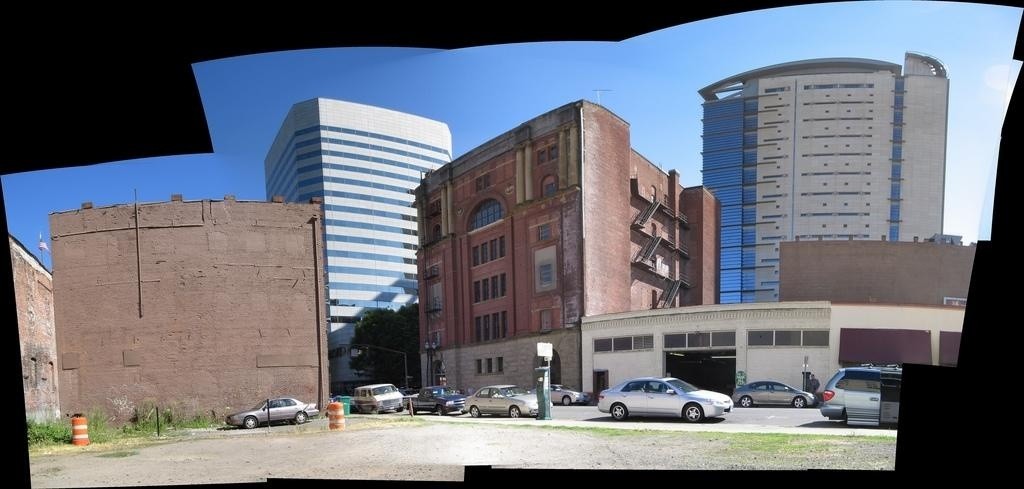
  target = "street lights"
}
[424,340,437,385]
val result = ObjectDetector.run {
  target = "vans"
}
[353,383,404,414]
[821,363,902,428]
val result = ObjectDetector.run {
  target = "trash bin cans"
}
[338,396,352,415]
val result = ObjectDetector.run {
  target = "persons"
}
[808,374,820,393]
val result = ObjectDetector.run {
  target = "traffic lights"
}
[352,345,370,350]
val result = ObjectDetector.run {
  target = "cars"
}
[731,380,818,408]
[598,376,734,423]
[226,397,320,429]
[464,383,590,418]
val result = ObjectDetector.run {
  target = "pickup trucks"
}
[403,385,467,416]
